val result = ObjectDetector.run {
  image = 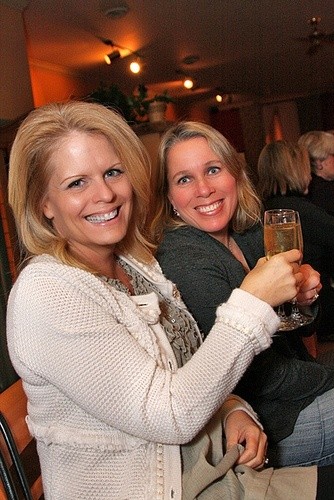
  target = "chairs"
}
[0,378,43,500]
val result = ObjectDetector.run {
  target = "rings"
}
[262,454,269,464]
[313,288,319,299]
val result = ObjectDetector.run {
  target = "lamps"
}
[104,50,121,65]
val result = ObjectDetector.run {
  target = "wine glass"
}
[264,209,299,331]
[286,211,315,327]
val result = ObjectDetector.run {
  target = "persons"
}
[4,99,334,499]
[251,130,334,345]
[150,119,334,471]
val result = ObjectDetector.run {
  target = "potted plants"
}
[126,84,178,122]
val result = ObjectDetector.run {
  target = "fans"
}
[293,17,334,56]
